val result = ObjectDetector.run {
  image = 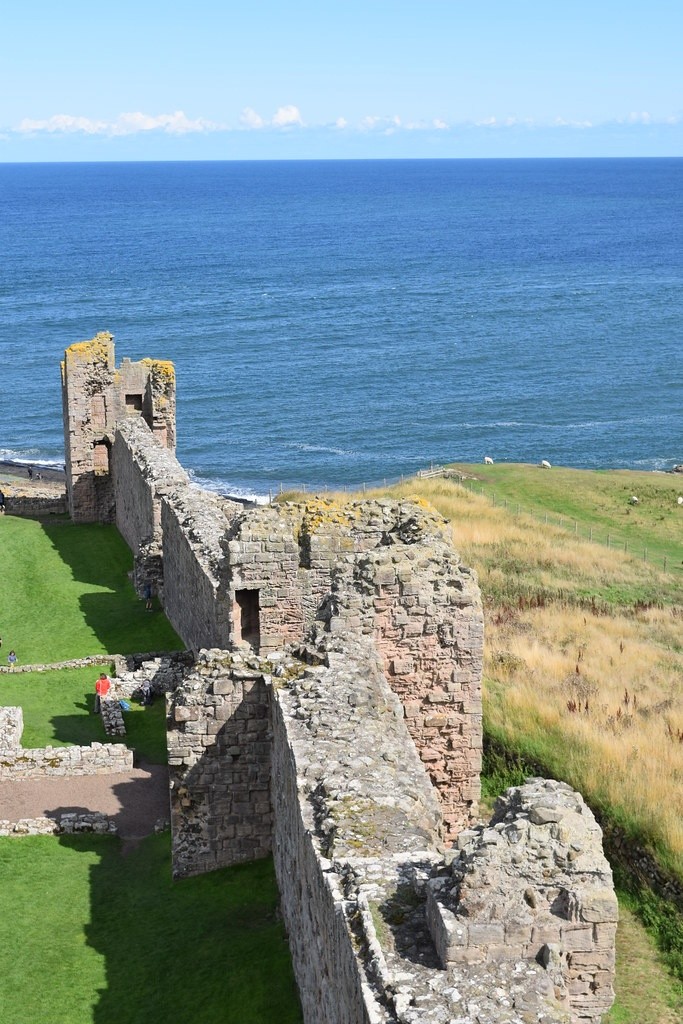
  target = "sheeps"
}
[541,459,553,469]
[484,456,495,465]
[630,496,683,506]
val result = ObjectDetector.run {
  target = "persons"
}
[143,583,154,612]
[7,651,18,667]
[28,467,33,481]
[0,490,4,512]
[36,473,41,479]
[93,673,110,714]
